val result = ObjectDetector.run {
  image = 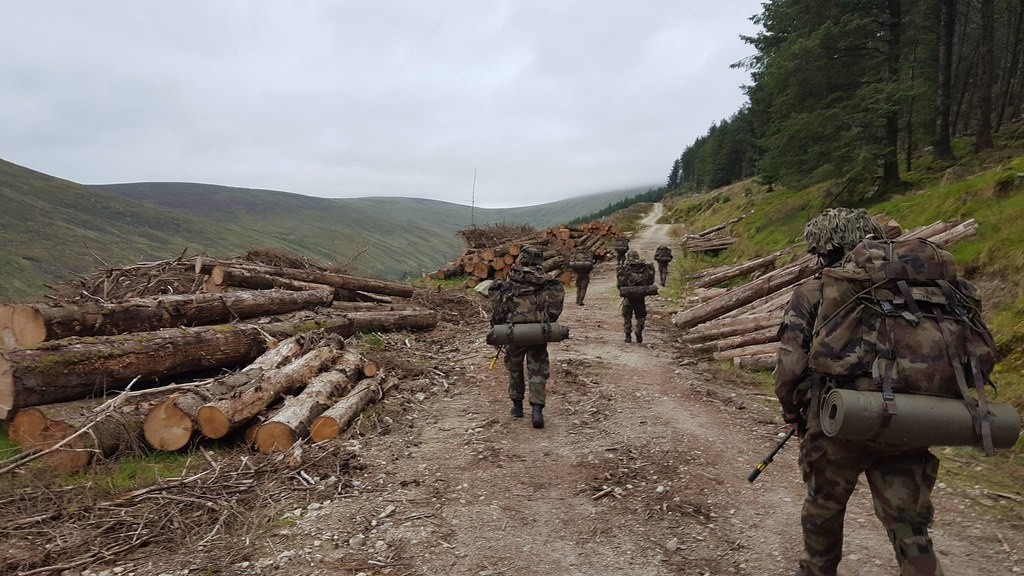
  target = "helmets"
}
[804,207,886,255]
[518,246,544,266]
[626,250,640,262]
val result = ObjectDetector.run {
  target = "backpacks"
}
[616,259,656,308]
[654,246,669,263]
[571,246,594,273]
[487,262,552,355]
[614,236,629,252]
[791,234,1000,458]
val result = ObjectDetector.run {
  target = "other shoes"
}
[624,336,632,343]
[530,412,545,427]
[575,299,585,306]
[660,281,665,288]
[510,405,524,418]
[634,328,643,343]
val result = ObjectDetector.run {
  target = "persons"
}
[615,237,629,265]
[772,207,998,576]
[654,246,673,287]
[617,251,656,343]
[490,246,566,428]
[569,247,593,305]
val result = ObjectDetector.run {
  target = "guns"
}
[489,347,502,371]
[747,428,796,484]
